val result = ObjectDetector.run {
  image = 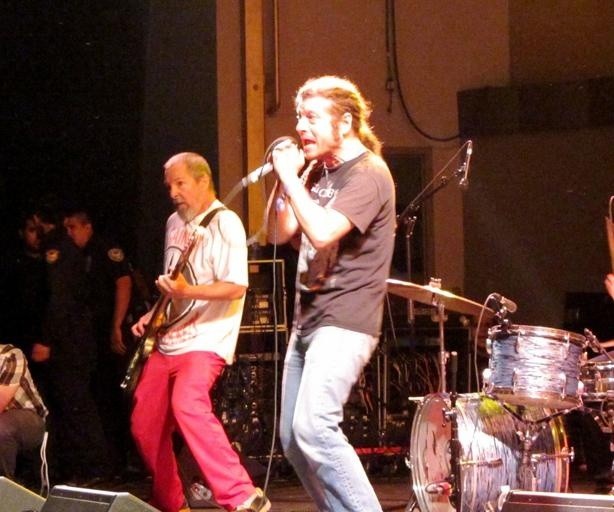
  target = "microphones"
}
[250,148,304,183]
[494,293,517,313]
[455,142,473,192]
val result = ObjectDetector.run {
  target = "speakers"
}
[521,80,592,129]
[0,476,46,512]
[40,485,161,512]
[591,75,614,127]
[563,291,614,343]
[459,88,522,139]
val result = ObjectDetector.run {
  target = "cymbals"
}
[385,277,492,318]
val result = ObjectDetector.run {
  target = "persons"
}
[604,194,613,301]
[266,77,399,511]
[126,150,275,511]
[0,199,146,489]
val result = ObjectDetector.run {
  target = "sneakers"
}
[234,486,271,511]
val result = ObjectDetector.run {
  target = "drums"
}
[586,361,614,402]
[410,391,575,512]
[483,324,587,412]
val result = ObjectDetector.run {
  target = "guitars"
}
[122,226,205,397]
